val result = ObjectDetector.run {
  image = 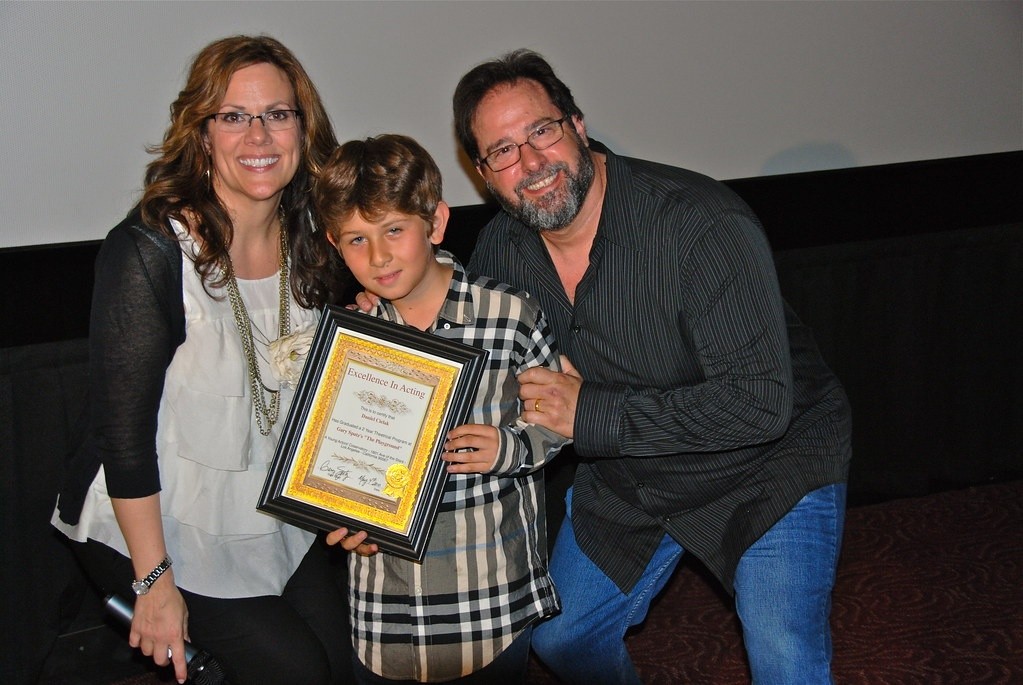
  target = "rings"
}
[535,398,541,412]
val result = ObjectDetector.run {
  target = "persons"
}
[316,135,576,685]
[452,49,854,685]
[48,37,353,684]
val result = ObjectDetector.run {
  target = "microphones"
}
[102,591,223,685]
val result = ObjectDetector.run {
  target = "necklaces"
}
[200,195,294,436]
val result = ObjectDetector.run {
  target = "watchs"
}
[131,554,173,596]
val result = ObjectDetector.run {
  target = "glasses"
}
[479,112,568,174]
[205,109,304,133]
